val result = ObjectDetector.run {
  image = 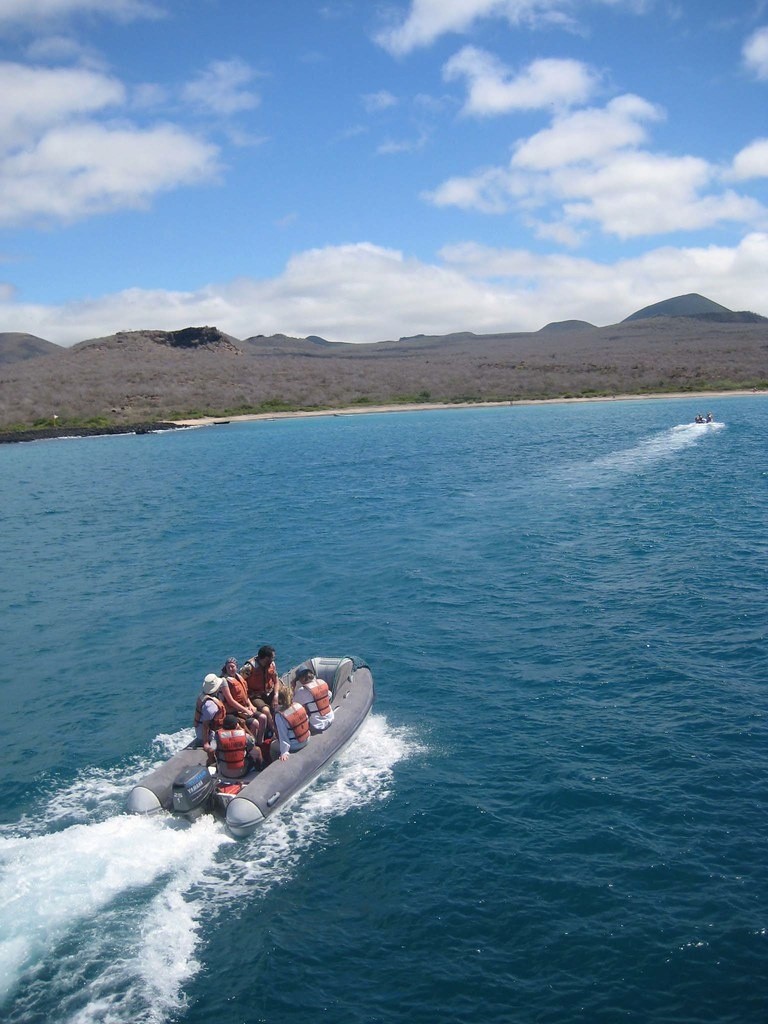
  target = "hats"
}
[293,665,313,683]
[226,657,238,668]
[222,715,239,728]
[202,674,222,694]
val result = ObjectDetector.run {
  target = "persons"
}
[194,645,335,780]
[695,411,713,422]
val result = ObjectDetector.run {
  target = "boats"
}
[127,654,375,838]
